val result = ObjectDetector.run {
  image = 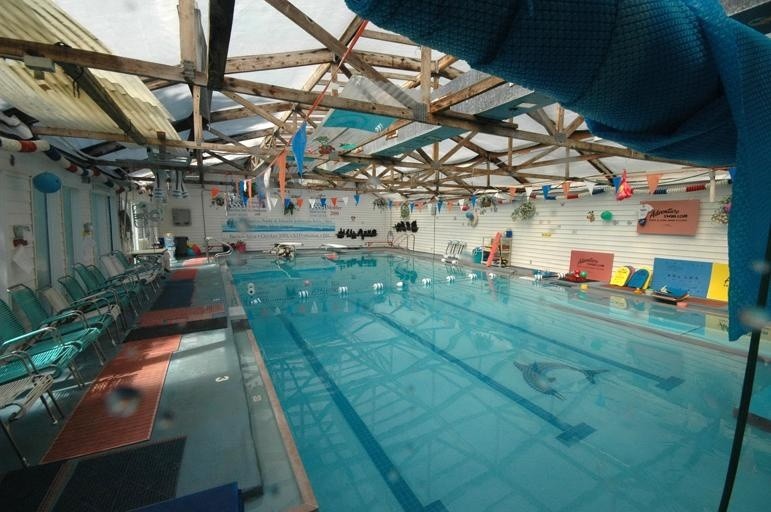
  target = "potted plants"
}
[474,193,499,215]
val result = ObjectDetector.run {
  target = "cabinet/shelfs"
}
[482,234,512,267]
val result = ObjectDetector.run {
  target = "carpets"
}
[182,253,212,266]
[149,276,194,310]
[116,316,230,342]
[53,427,188,511]
[172,268,199,280]
[107,333,186,358]
[137,303,230,325]
[598,280,649,294]
[0,462,59,511]
[686,291,732,309]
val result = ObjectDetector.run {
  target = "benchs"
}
[364,240,393,248]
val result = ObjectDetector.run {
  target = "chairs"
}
[1,244,173,470]
